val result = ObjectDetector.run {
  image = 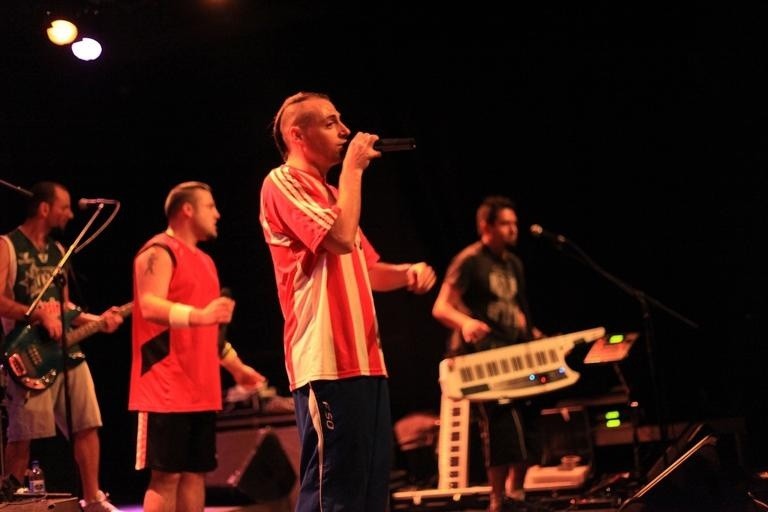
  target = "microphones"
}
[218,287,231,352]
[342,138,416,154]
[531,222,567,244]
[77,196,118,210]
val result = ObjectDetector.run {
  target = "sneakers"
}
[79,491,120,511]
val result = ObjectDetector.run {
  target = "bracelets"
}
[168,301,193,331]
[220,342,237,367]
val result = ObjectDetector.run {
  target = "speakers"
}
[641,419,717,482]
[204,412,300,507]
[618,434,733,512]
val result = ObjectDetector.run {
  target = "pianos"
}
[437,327,606,403]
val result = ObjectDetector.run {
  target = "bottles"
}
[29,460,45,498]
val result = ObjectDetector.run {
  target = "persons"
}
[0,181,120,512]
[425,193,548,512]
[127,180,267,512]
[258,88,437,512]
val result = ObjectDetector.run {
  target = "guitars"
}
[2,301,132,392]
